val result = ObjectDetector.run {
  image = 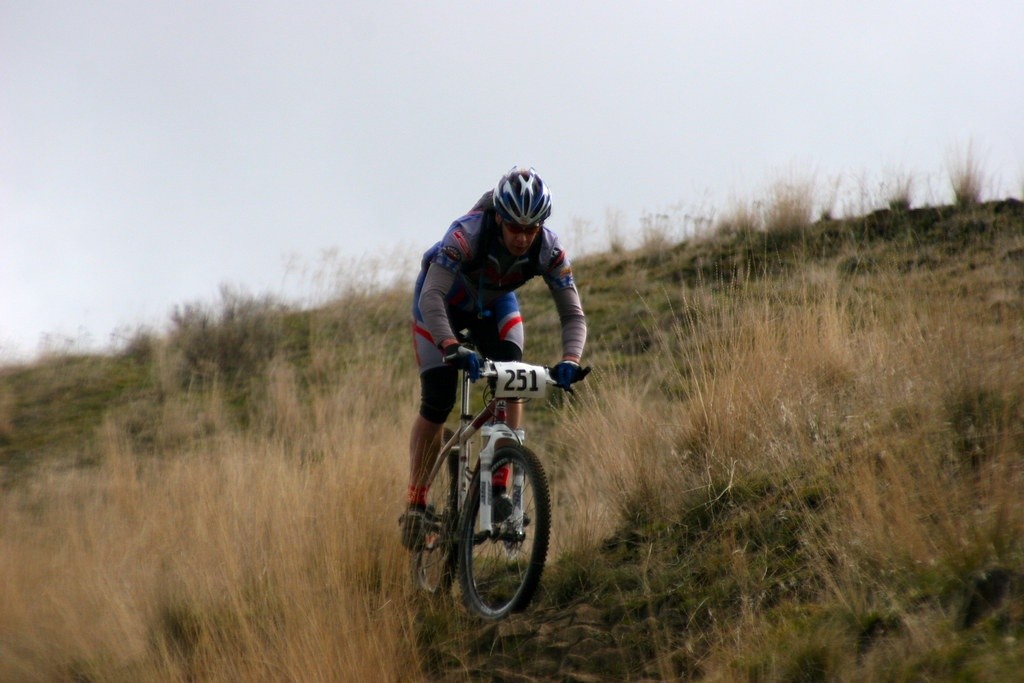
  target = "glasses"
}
[502,217,541,234]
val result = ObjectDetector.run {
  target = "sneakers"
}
[493,493,531,527]
[398,503,426,550]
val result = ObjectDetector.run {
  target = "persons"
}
[401,164,587,551]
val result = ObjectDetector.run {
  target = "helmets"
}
[492,165,552,227]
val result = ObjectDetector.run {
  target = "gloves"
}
[446,342,485,383]
[549,359,579,391]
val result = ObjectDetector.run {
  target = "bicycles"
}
[405,329,594,620]
